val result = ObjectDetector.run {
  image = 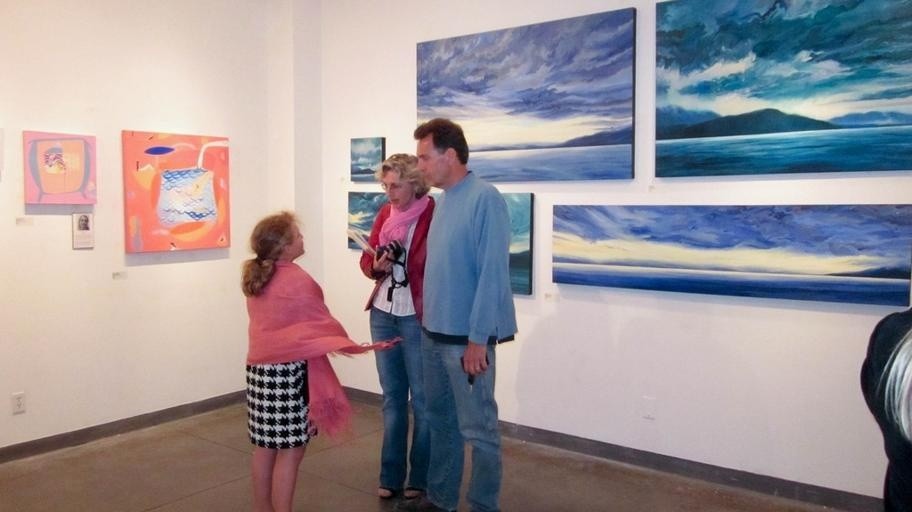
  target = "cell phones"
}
[461,354,490,372]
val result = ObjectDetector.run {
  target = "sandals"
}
[378,486,396,499]
[403,487,424,500]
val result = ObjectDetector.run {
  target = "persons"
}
[359,153,435,500]
[413,118,519,512]
[241,211,401,512]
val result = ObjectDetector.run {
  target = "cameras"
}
[376,239,402,264]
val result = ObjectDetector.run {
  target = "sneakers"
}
[396,499,450,512]
